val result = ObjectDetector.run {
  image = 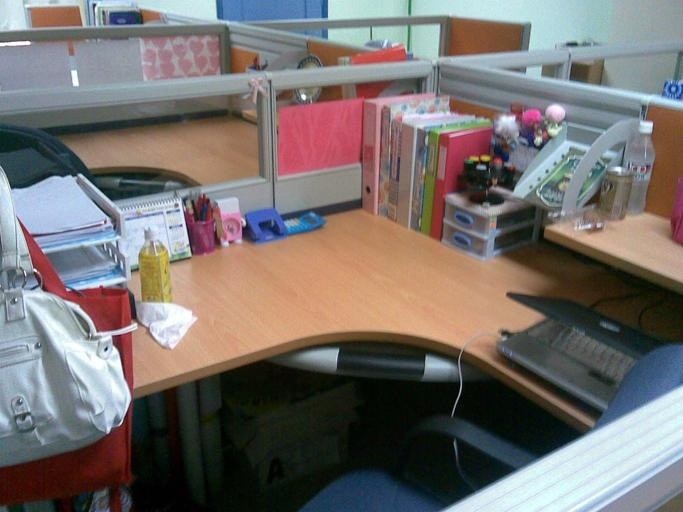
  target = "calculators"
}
[284,210,326,234]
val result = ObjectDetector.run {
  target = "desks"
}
[132,209,679,436]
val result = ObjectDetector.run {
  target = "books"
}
[338,44,414,102]
[522,144,613,213]
[8,173,120,292]
[359,88,495,242]
[78,0,146,28]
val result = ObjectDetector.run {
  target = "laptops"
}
[496,292,669,411]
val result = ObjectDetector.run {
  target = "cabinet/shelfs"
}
[542,113,682,291]
[35,175,130,293]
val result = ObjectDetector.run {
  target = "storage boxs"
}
[441,188,538,255]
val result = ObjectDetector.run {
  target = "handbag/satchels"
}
[0,285,132,468]
[0,216,137,512]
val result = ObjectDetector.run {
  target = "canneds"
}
[598,166,633,221]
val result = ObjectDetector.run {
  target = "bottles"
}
[620,120,656,215]
[138,228,171,306]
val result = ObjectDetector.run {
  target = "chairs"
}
[300,342,679,509]
[1,121,97,190]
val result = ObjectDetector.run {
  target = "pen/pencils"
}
[183,190,214,222]
